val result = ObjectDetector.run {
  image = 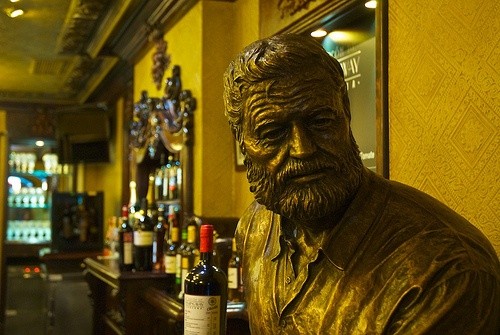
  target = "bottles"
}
[184,224,228,335]
[213,231,244,302]
[103,160,200,291]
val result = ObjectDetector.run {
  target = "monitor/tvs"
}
[53,102,112,144]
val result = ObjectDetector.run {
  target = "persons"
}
[221,34,500,335]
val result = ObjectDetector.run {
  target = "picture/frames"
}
[275,0,389,183]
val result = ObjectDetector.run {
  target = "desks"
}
[80,258,249,335]
